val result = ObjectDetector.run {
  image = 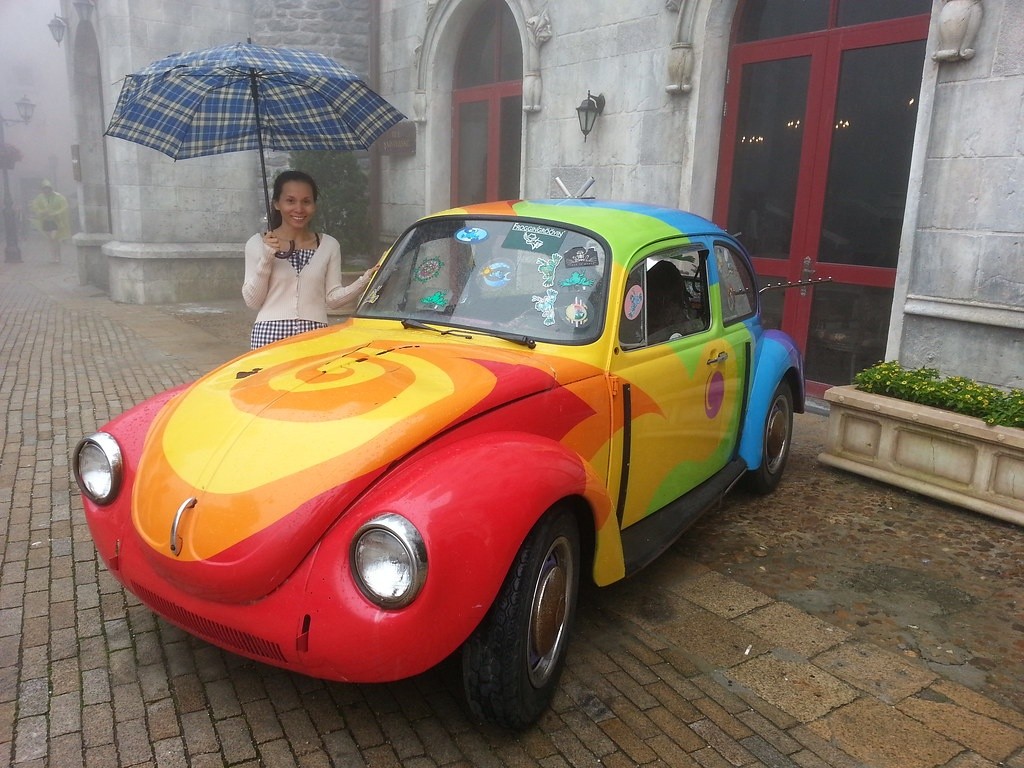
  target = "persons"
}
[32,180,68,263]
[242,171,382,353]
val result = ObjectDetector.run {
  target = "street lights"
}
[1,95,37,265]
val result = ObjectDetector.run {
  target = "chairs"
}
[620,260,697,340]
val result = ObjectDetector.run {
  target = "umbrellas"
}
[102,31,408,259]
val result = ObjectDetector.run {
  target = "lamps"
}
[574,88,610,143]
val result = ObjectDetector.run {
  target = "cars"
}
[74,195,805,734]
